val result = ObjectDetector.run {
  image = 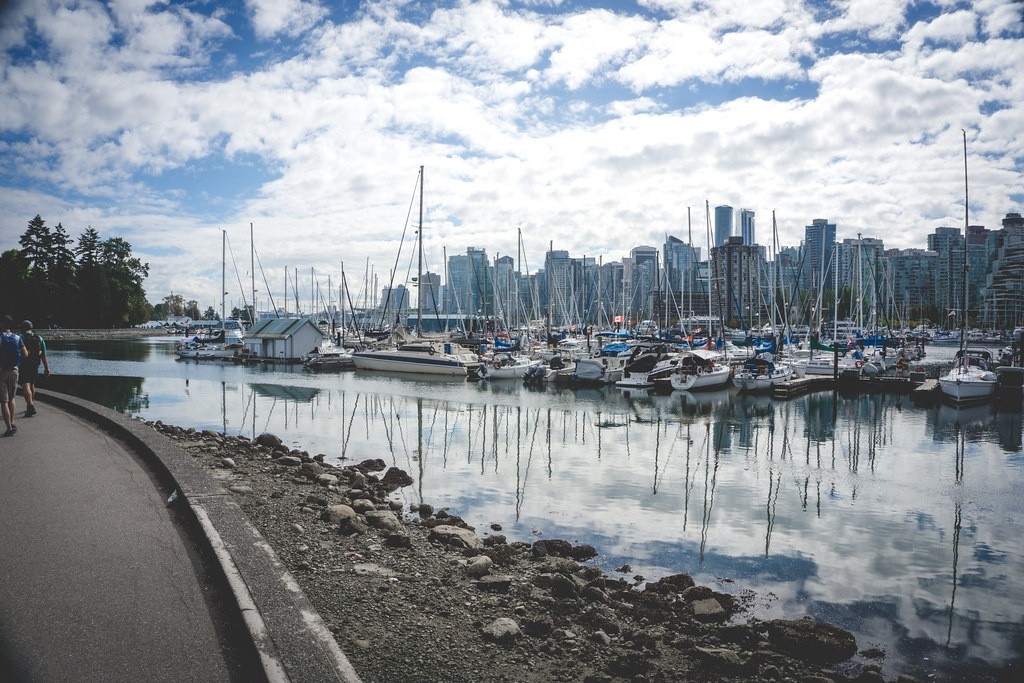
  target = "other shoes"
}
[3,423,18,437]
[22,404,37,418]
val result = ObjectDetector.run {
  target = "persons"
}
[0,314,50,436]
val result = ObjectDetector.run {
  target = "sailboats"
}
[940,129,1000,406]
[174,230,244,360]
[351,166,478,376]
[441,199,1023,408]
[132,243,441,371]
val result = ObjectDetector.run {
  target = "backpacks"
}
[18,331,40,366]
[0,332,18,367]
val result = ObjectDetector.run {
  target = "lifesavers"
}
[493,361,501,369]
[855,361,863,368]
[428,346,435,356]
[697,366,703,375]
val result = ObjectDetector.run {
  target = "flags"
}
[695,328,702,332]
[571,323,576,330]
[682,335,692,341]
[614,314,624,322]
